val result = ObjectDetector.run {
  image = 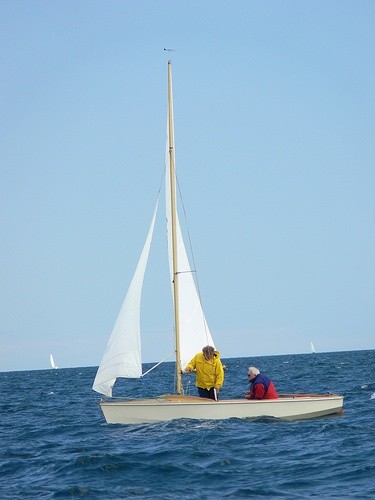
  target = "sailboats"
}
[90,59,347,426]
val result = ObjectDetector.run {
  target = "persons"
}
[244,366,279,400]
[186,344,224,401]
